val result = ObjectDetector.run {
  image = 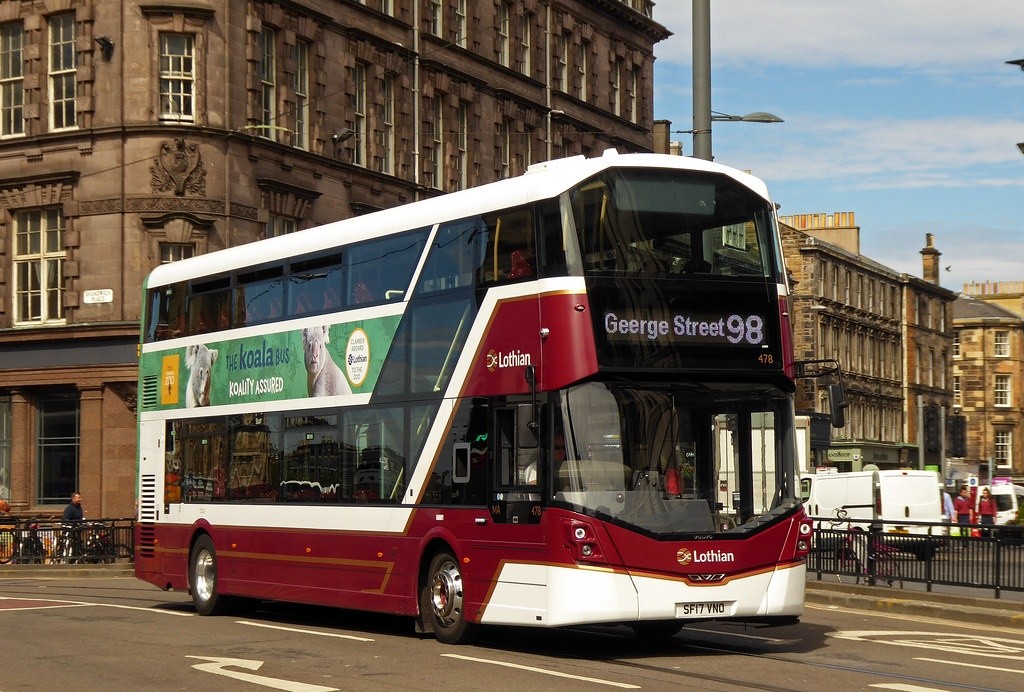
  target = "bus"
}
[134,148,849,644]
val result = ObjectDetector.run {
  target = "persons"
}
[943,492,955,545]
[978,487,997,548]
[442,430,566,496]
[62,492,83,556]
[954,488,975,548]
[21,519,44,563]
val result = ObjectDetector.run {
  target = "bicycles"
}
[0,512,132,564]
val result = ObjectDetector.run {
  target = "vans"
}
[975,481,1024,534]
[777,468,944,561]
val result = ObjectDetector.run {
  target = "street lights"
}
[692,112,786,163]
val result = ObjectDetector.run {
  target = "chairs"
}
[156,248,536,340]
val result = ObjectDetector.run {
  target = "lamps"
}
[92,34,115,50]
[332,127,357,143]
